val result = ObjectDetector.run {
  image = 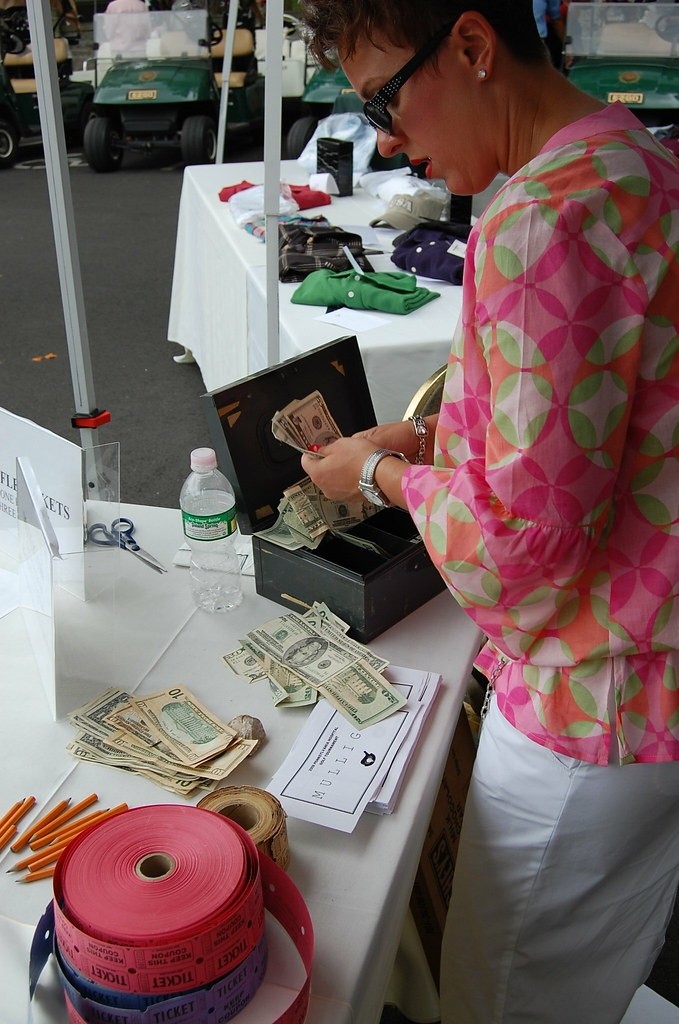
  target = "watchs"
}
[357,449,409,509]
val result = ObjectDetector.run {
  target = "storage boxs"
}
[199,335,446,646]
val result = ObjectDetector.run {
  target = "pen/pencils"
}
[0,794,129,884]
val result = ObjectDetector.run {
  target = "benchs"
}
[5,28,254,93]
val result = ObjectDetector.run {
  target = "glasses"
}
[363,21,457,135]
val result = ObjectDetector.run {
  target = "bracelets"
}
[408,413,429,465]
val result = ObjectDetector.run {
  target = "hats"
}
[369,193,443,230]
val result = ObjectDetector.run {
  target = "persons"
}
[298,0,679,1024]
[532,0,563,68]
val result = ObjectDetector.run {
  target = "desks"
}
[165,159,466,431]
[3,502,479,1024]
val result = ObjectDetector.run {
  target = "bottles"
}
[180,447,244,613]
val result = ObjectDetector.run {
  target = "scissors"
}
[88,518,168,574]
